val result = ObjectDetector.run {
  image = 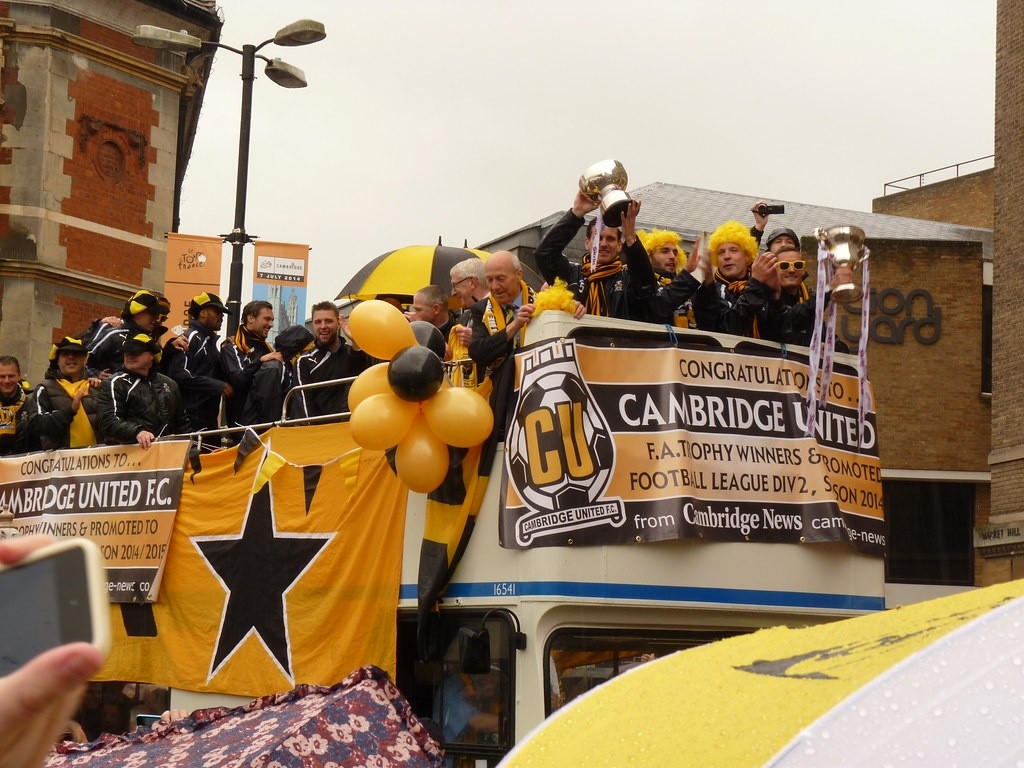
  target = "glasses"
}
[449,276,478,290]
[777,260,806,272]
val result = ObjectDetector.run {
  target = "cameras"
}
[757,202,784,217]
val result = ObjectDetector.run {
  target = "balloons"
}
[348,299,493,495]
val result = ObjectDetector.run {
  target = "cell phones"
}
[0,538,111,677]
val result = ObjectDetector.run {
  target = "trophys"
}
[812,226,870,304]
[578,158,639,228]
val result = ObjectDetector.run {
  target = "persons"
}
[290,301,370,427]
[692,219,778,351]
[220,300,283,446]
[749,200,859,378]
[98,333,193,450]
[73,289,189,375]
[28,336,104,450]
[0,533,103,767]
[168,291,235,455]
[532,190,665,348]
[71,681,170,743]
[382,251,586,442]
[0,355,42,457]
[434,660,499,768]
[634,227,702,343]
[240,324,315,437]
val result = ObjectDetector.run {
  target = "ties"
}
[506,305,516,357]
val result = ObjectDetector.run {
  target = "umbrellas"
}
[491,578,1024,768]
[333,235,545,315]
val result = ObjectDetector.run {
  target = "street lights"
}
[133,20,326,428]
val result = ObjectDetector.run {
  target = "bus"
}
[2,307,887,768]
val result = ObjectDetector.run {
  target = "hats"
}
[766,227,800,250]
[188,291,233,317]
[121,289,158,318]
[116,331,161,355]
[18,379,34,395]
[48,335,89,365]
